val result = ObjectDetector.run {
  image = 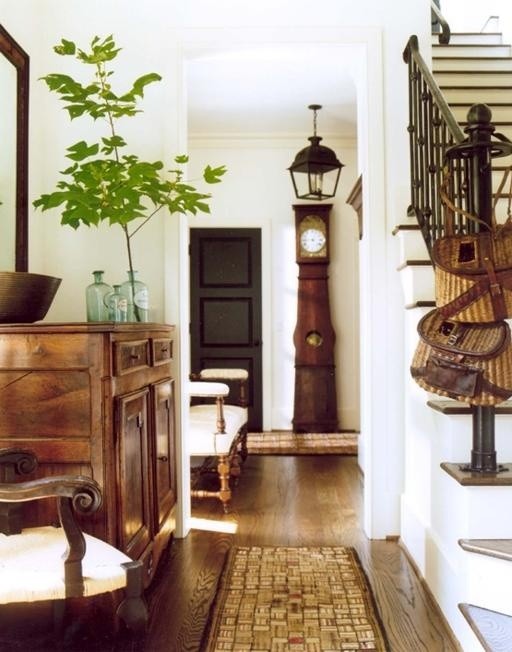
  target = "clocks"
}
[292,204,337,433]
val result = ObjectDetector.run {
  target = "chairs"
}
[189,368,249,515]
[0,452,149,652]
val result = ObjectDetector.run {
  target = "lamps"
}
[286,105,345,200]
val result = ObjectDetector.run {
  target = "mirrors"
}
[0,25,30,275]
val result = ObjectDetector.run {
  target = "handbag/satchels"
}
[430,227,512,323]
[409,308,512,408]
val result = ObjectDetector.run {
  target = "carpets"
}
[197,545,392,652]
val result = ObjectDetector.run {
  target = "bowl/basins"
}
[0,271,64,324]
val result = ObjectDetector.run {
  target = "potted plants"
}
[34,34,228,323]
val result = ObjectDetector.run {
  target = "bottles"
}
[85,269,113,322]
[106,284,127,322]
[123,270,150,322]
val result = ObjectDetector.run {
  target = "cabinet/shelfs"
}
[0,321,178,633]
